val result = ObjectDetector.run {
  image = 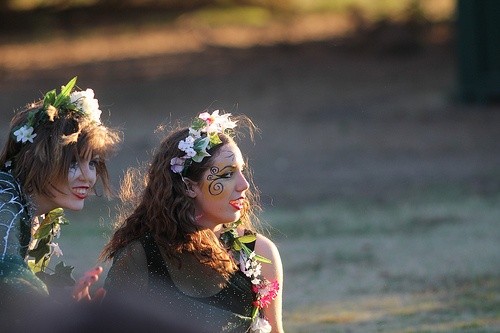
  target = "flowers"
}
[44,216,65,256]
[15,82,102,143]
[239,252,281,333]
[168,110,237,173]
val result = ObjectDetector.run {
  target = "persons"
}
[103,110,283,333]
[1,76,124,333]
